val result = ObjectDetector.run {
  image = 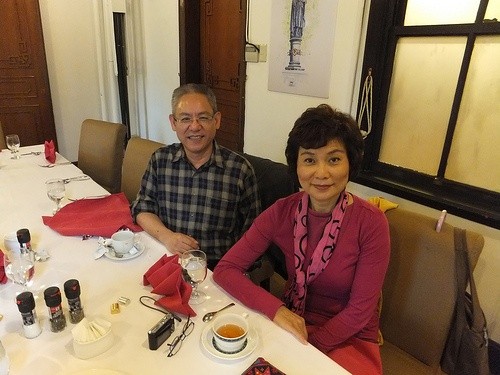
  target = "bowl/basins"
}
[3,233,19,251]
[72,328,113,360]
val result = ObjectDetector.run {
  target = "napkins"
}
[44,140,56,163]
[42,192,144,237]
[143,253,196,317]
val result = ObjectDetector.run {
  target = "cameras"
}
[148,313,175,350]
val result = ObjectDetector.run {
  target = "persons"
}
[213,102,391,375]
[131,84,263,272]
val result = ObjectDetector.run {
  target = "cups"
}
[212,312,249,353]
[108,230,134,255]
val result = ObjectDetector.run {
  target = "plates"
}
[201,324,259,360]
[104,242,145,260]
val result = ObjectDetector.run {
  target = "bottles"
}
[16,292,42,339]
[17,229,35,262]
[44,287,66,333]
[63,279,84,325]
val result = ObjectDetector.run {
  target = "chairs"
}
[379,208,484,374]
[237,151,295,295]
[78,118,127,194]
[121,136,167,204]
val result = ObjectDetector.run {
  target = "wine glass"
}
[44,179,66,216]
[181,250,207,305]
[6,134,21,160]
[4,248,35,292]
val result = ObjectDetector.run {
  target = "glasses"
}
[173,115,214,126]
[167,313,195,357]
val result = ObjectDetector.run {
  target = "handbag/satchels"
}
[440,227,490,375]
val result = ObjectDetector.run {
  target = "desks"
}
[0,144,351,375]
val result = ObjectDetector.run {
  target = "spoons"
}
[202,302,236,322]
[107,245,123,258]
